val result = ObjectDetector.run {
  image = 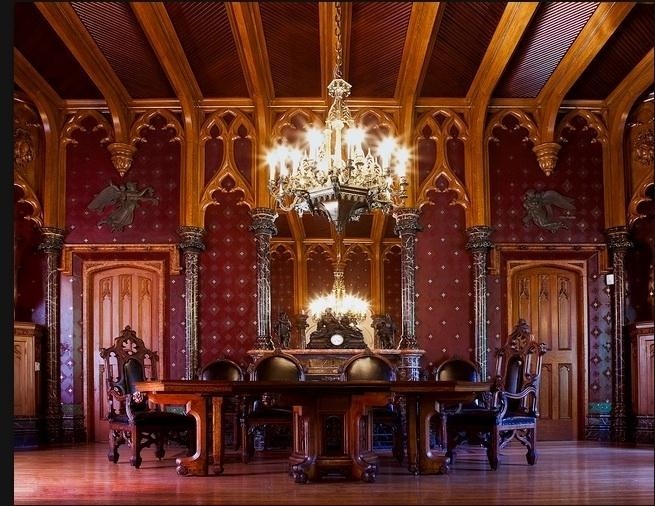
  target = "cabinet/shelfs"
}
[14,321,45,450]
[628,320,655,445]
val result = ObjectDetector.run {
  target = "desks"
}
[135,379,493,483]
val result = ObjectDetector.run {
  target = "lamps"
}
[267,2,409,237]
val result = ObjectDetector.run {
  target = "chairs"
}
[100,325,194,469]
[198,352,243,451]
[445,318,547,471]
[240,348,305,464]
[341,346,405,462]
[436,353,488,448]
